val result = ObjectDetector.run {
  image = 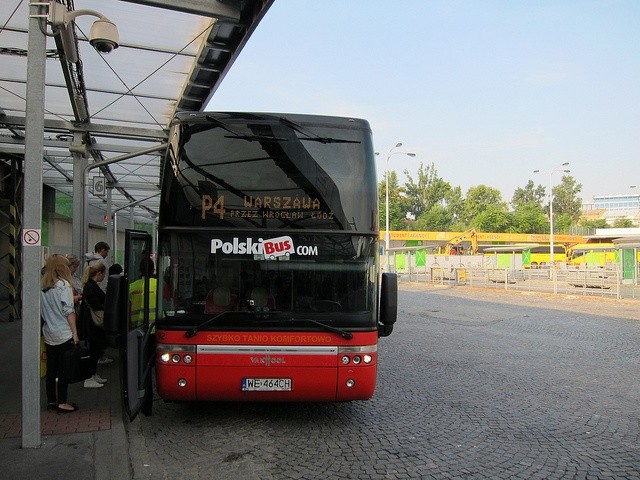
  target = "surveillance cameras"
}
[32,0,129,63]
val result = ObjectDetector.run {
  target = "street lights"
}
[374,140,417,270]
[628,183,638,191]
[532,159,572,279]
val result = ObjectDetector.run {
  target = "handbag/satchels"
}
[63,348,99,384]
[41,336,47,378]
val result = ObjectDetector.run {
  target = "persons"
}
[76,263,108,388]
[128,258,158,417]
[40,254,82,414]
[82,242,114,364]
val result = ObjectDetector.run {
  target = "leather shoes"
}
[47,404,56,409]
[56,407,78,414]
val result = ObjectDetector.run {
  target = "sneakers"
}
[84,377,104,387]
[92,373,107,383]
[98,359,107,364]
[103,356,113,362]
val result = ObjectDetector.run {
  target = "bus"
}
[483,244,566,283]
[566,243,640,289]
[119,111,398,423]
